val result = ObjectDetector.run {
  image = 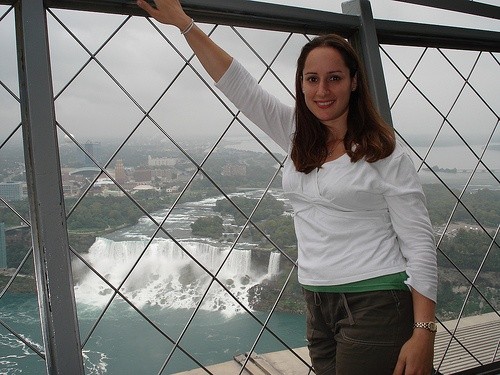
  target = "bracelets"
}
[181,18,195,35]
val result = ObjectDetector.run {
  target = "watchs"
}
[413,321,438,333]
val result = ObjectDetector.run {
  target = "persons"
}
[136,0,438,375]
